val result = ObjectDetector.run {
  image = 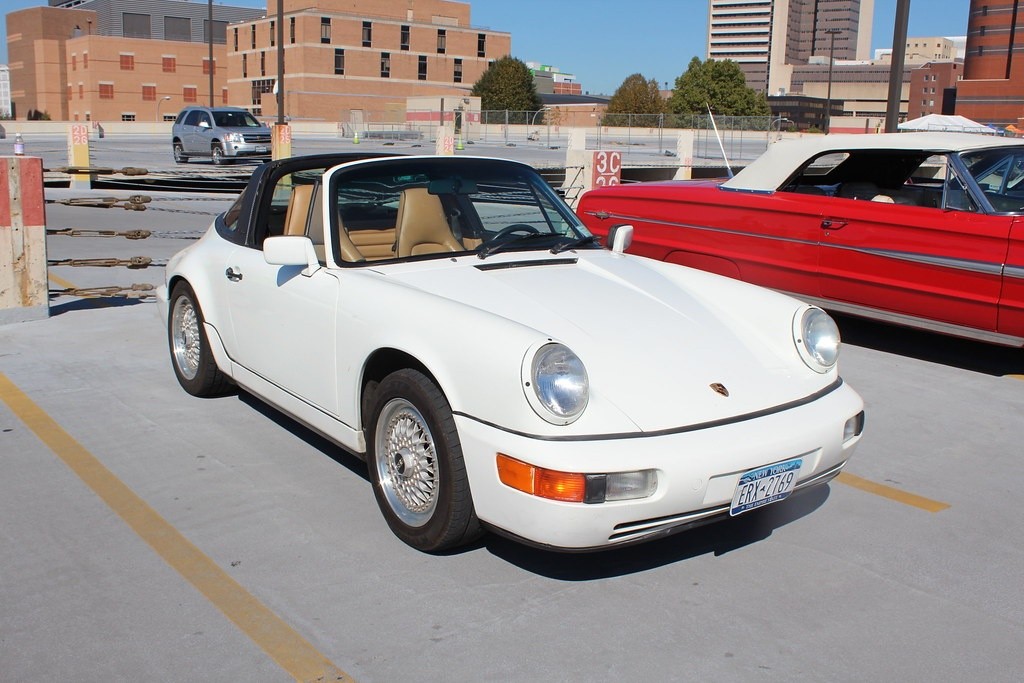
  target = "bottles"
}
[14,133,24,155]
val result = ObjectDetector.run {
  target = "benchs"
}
[347,228,397,262]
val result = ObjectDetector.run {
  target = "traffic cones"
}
[353,131,360,144]
[456,132,466,151]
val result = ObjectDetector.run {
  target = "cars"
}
[574,129,1024,365]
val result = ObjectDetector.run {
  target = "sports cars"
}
[154,150,867,556]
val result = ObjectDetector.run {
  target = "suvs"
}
[172,103,273,165]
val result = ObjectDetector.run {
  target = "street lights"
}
[825,28,843,134]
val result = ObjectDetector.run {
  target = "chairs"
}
[394,188,465,256]
[283,185,366,264]
[837,181,878,196]
